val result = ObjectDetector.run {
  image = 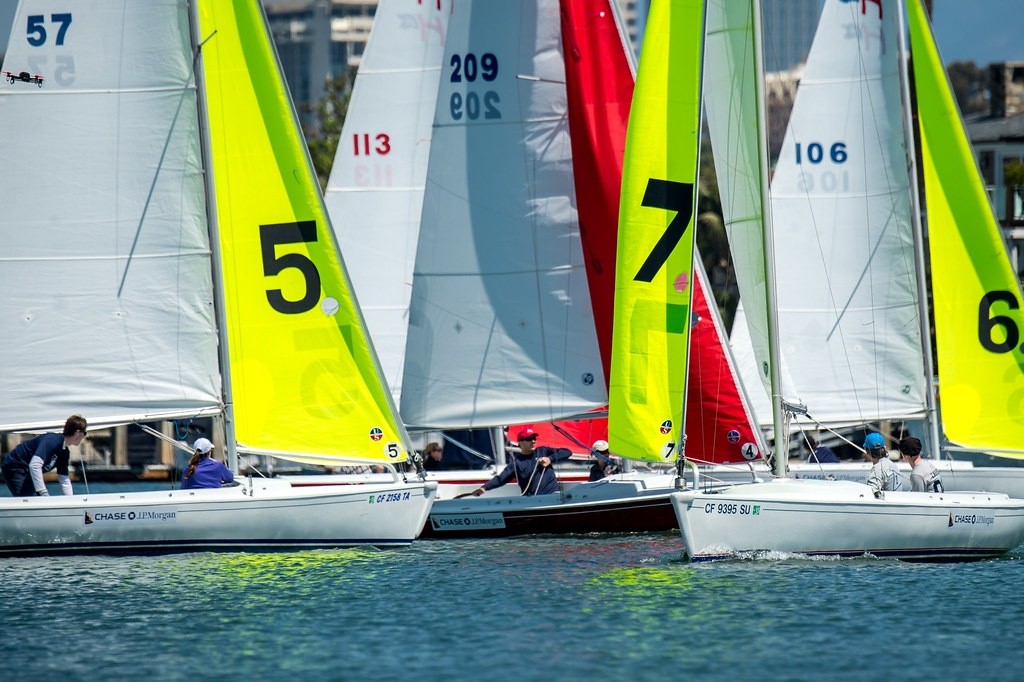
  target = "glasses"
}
[520,437,536,442]
[80,431,87,436]
[434,448,442,452]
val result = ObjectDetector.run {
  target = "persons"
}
[370,460,417,473]
[899,437,944,493]
[864,433,902,492]
[4,416,87,496]
[473,429,572,496]
[180,438,233,489]
[590,440,619,480]
[804,436,841,463]
[425,443,448,470]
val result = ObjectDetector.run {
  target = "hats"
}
[518,429,538,442]
[194,438,215,454]
[803,436,815,448]
[863,433,885,448]
[592,440,609,452]
[899,437,923,456]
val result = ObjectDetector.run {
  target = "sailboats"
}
[608,0,1024,562]
[284,402,974,493]
[0,0,440,549]
[321,1,768,545]
